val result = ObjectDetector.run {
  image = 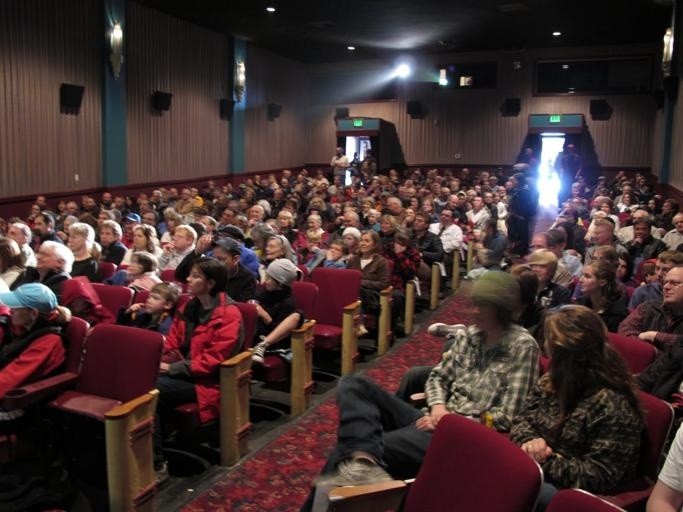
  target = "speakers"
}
[60,83,85,115]
[590,100,612,120]
[336,107,349,117]
[270,104,282,117]
[220,99,235,119]
[153,91,172,110]
[407,101,418,113]
[503,98,521,115]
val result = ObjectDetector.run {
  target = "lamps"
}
[107,21,126,77]
[233,60,249,104]
[657,5,676,77]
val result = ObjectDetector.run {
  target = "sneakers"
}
[316,463,393,493]
[426,322,467,339]
[251,342,267,363]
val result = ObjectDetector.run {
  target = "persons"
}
[329,146,348,188]
[0,162,682,509]
[553,143,585,214]
[512,145,537,180]
[360,150,377,166]
[357,155,377,183]
[346,152,362,181]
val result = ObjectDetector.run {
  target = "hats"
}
[342,226,362,241]
[266,258,299,285]
[124,212,141,223]
[0,282,58,311]
